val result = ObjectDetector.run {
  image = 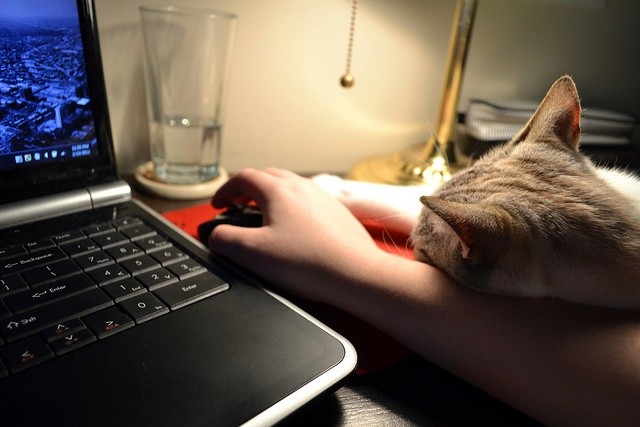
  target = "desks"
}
[122,168,528,426]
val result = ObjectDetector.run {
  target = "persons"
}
[208,167,640,427]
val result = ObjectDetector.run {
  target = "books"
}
[466,96,636,143]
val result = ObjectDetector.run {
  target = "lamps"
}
[338,1,482,184]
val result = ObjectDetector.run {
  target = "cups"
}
[138,5,236,183]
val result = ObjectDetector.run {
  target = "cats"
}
[309,72,640,315]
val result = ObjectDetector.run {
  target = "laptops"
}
[0,0,358,427]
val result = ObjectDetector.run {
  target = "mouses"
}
[197,203,262,248]
